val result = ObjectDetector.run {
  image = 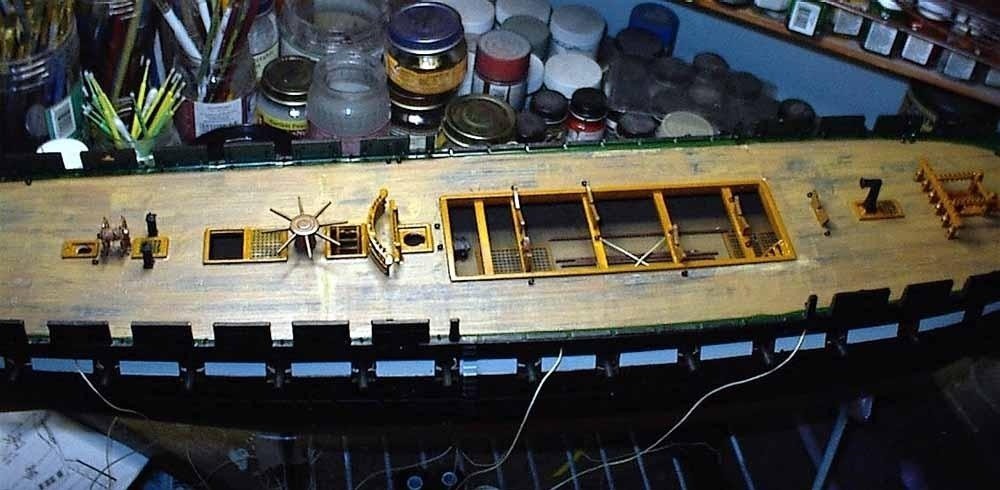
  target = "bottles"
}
[0,0,817,154]
[716,0,1000,88]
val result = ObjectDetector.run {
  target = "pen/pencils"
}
[0,0,74,62]
[79,54,187,156]
[88,0,148,62]
[153,0,260,102]
